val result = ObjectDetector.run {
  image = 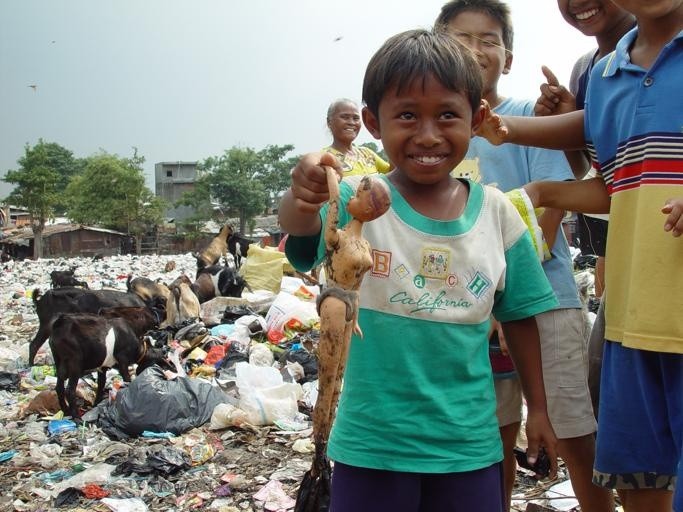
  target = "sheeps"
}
[27,226,256,418]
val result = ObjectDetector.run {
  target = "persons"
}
[435,1,683,512]
[293,163,391,512]
[314,95,396,180]
[275,27,562,512]
